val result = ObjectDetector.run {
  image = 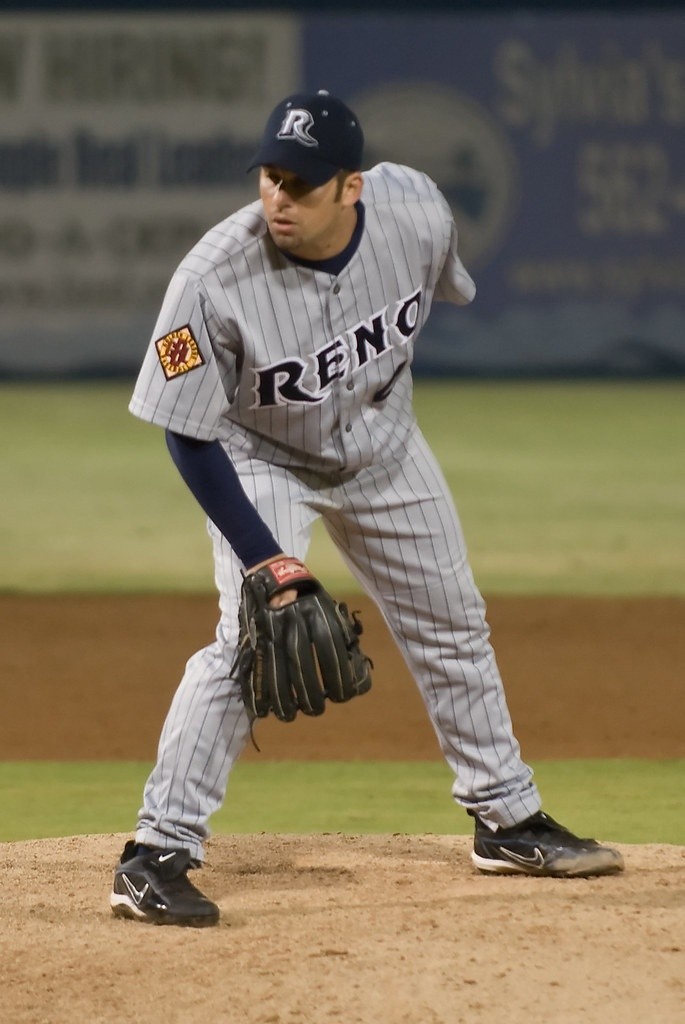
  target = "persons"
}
[107,90,626,928]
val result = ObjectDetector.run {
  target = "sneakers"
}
[109,840,220,927]
[467,808,624,876]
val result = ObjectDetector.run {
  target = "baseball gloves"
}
[237,558,371,723]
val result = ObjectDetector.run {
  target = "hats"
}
[244,89,364,184]
